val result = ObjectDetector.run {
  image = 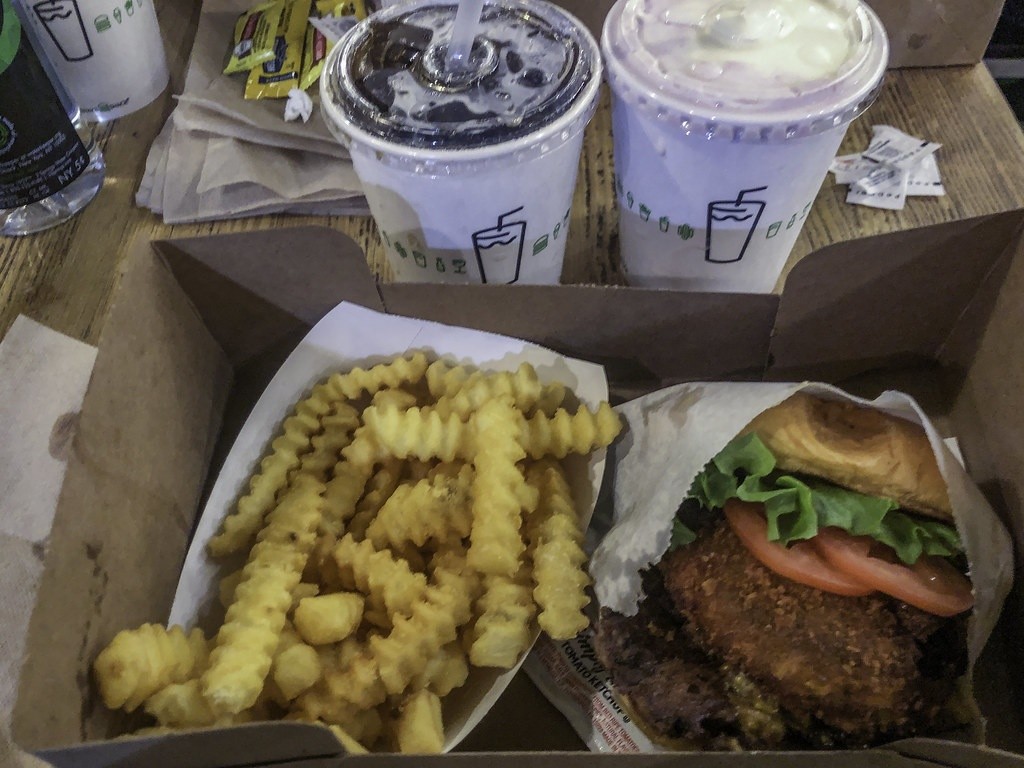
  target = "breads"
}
[732,390,958,525]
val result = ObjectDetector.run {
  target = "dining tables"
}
[1,0,1023,768]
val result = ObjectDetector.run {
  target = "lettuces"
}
[664,432,970,569]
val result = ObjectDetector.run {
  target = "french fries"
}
[93,350,623,754]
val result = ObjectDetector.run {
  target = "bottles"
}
[0,1,110,237]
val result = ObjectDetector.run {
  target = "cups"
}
[14,0,170,125]
[315,0,602,287]
[592,0,893,297]
